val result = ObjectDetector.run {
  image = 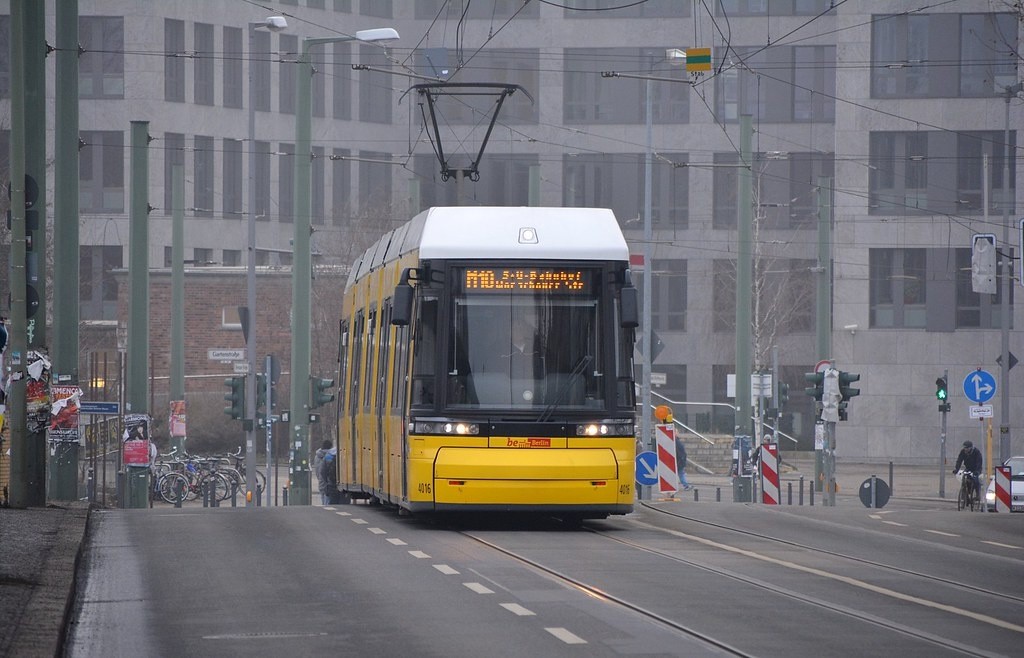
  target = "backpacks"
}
[328,456,336,483]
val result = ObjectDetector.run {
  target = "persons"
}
[675,429,691,490]
[752,434,781,476]
[135,423,147,440]
[953,441,982,500]
[314,439,351,505]
[496,318,546,379]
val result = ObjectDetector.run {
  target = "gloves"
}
[975,471,980,477]
[952,469,958,475]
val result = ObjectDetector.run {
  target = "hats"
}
[764,434,770,442]
[963,441,973,447]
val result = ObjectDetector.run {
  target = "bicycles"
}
[150,446,265,504]
[956,471,981,512]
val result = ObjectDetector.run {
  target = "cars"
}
[985,456,1024,512]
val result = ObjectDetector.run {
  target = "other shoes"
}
[960,500,965,508]
[685,485,692,490]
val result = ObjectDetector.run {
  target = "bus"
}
[333,205,641,523]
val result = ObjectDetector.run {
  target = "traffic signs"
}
[635,451,658,486]
[962,370,997,403]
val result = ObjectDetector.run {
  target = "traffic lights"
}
[308,374,335,408]
[805,372,823,402]
[936,377,948,400]
[838,371,860,401]
[224,376,245,420]
